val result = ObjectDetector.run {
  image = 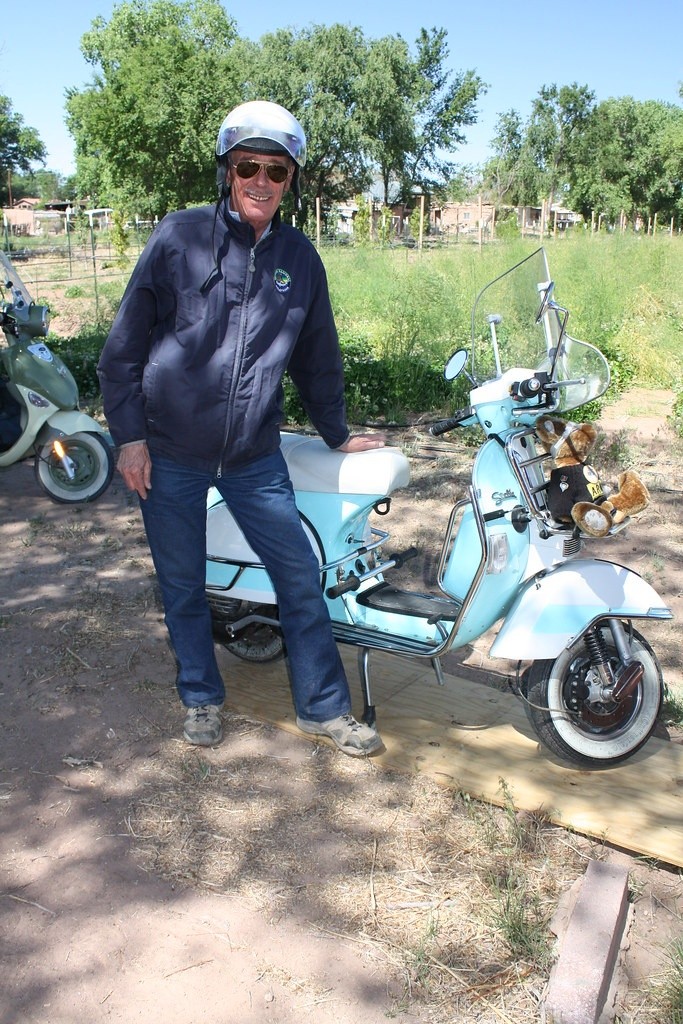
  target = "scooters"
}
[205,246,677,768]
[0,248,115,505]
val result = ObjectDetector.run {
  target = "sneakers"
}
[183,701,226,746]
[295,715,382,755]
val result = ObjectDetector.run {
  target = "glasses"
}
[226,157,291,183]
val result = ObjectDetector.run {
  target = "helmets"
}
[214,100,307,196]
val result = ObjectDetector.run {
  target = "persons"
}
[96,101,387,758]
